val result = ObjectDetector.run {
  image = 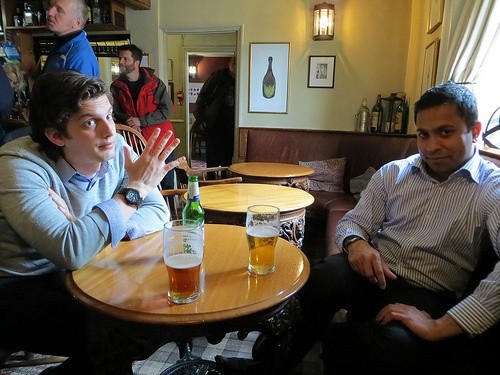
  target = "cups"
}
[163,219,204,305]
[245,205,281,276]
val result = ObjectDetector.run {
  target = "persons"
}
[44,0,100,77]
[0,69,188,375]
[195,56,237,179]
[110,45,177,212]
[216,82,500,375]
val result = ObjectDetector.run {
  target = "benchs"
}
[245,128,500,296]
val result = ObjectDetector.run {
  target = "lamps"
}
[313,2,335,41]
[189,63,196,77]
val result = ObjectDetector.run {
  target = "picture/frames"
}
[189,83,205,103]
[427,0,444,34]
[247,42,290,114]
[168,59,173,81]
[168,83,174,104]
[308,55,336,88]
[419,37,439,97]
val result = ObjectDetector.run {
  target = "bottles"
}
[182,175,205,256]
[394,93,408,134]
[370,94,383,133]
[13,0,111,26]
[354,97,369,132]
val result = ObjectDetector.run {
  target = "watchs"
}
[342,237,361,254]
[117,188,144,209]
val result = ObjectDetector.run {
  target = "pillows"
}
[299,157,347,192]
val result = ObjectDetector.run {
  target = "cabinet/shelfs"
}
[0,0,152,43]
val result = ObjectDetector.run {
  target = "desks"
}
[65,224,310,375]
[182,182,316,249]
[229,161,315,193]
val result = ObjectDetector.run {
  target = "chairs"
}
[114,124,243,221]
